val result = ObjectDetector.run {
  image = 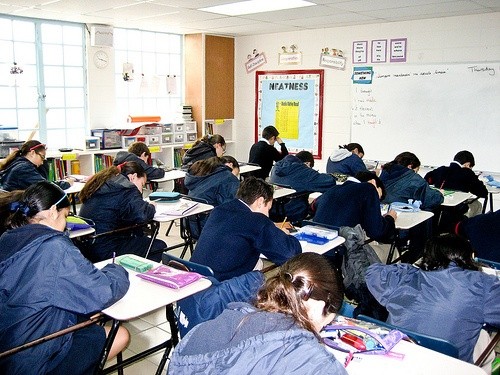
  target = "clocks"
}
[93,50,109,69]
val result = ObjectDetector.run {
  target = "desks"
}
[51,174,92,215]
[289,226,346,288]
[367,164,383,178]
[379,204,434,265]
[145,195,215,260]
[64,226,96,249]
[149,166,187,193]
[482,183,500,214]
[432,188,473,237]
[93,253,212,375]
[268,183,297,223]
[481,267,500,276]
[239,165,262,187]
[319,314,488,375]
[308,170,361,205]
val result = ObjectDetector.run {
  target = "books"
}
[174,149,187,167]
[94,155,114,173]
[175,177,189,195]
[46,157,68,180]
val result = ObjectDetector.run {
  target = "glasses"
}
[35,152,47,161]
[218,142,226,154]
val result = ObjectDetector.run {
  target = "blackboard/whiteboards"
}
[348,59,499,177]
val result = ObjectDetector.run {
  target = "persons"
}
[327,142,380,181]
[364,233,500,375]
[168,252,348,375]
[249,126,289,182]
[268,150,336,222]
[182,134,226,168]
[379,151,444,263]
[113,143,165,180]
[0,181,130,375]
[423,150,488,233]
[313,170,401,265]
[190,176,302,281]
[0,140,73,191]
[79,161,168,262]
[452,210,500,263]
[184,156,241,238]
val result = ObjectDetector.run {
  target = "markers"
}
[374,160,384,162]
[424,165,434,167]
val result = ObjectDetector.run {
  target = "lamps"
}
[122,62,133,81]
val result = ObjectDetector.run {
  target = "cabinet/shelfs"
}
[203,119,236,160]
[0,142,201,205]
[122,121,198,147]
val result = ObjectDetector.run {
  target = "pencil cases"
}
[161,200,199,216]
[135,265,203,289]
[391,201,419,213]
[294,224,339,244]
[148,191,181,203]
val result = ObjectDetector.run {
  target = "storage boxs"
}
[182,106,193,121]
[90,129,122,150]
[84,137,100,151]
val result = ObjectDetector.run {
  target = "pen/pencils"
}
[476,171,483,178]
[439,181,446,191]
[66,172,71,180]
[317,169,319,171]
[149,197,162,203]
[320,315,406,355]
[340,353,354,369]
[387,204,391,213]
[113,252,116,263]
[158,162,161,168]
[374,161,380,170]
[279,216,287,231]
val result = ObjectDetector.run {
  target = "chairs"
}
[301,219,410,265]
[172,194,209,259]
[0,314,104,375]
[238,161,261,167]
[355,314,500,367]
[469,256,500,270]
[440,193,489,243]
[155,253,283,375]
